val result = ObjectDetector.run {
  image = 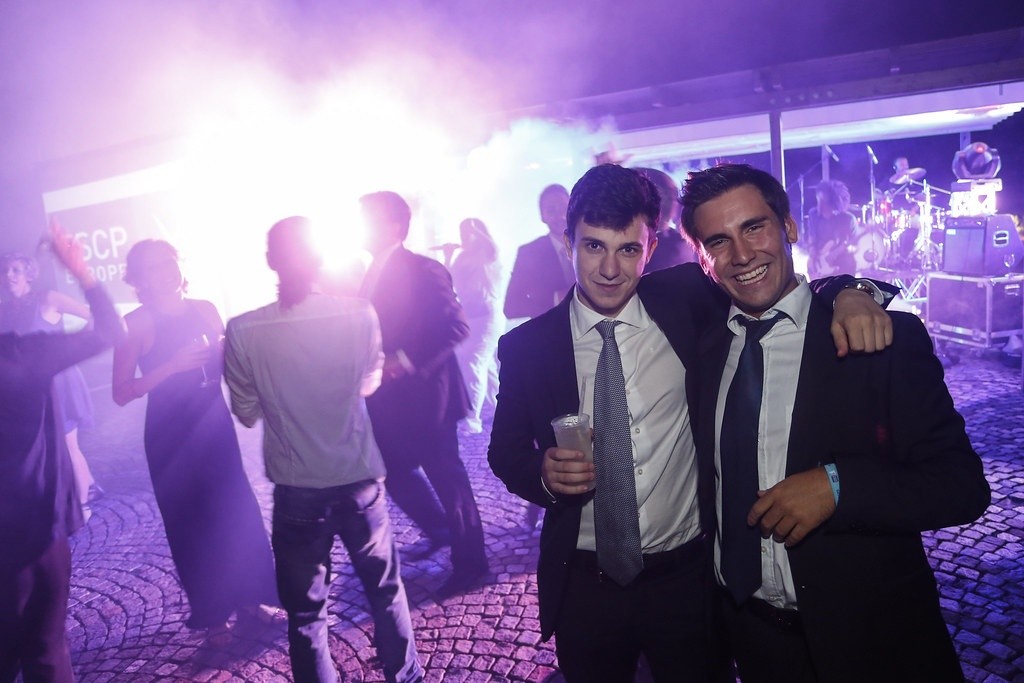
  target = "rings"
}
[67,244,71,248]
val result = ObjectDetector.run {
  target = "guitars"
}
[806,222,883,281]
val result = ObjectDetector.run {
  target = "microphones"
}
[824,144,839,163]
[867,144,878,164]
[431,243,460,251]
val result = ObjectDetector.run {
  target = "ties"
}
[720,311,783,595]
[558,246,574,285]
[593,319,643,587]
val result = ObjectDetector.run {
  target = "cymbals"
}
[889,167,926,184]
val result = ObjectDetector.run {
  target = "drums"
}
[854,226,891,271]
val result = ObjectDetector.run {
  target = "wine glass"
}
[188,333,220,388]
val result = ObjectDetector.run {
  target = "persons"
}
[357,192,496,595]
[680,164,993,683]
[111,240,290,645]
[505,165,861,323]
[487,164,892,683]
[0,213,126,683]
[222,217,424,683]
[442,217,498,434]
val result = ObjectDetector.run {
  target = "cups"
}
[550,413,598,494]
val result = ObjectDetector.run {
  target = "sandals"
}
[236,605,286,626]
[206,622,230,643]
[80,483,106,526]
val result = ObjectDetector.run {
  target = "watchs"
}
[832,283,874,310]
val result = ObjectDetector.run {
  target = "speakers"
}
[941,213,1024,275]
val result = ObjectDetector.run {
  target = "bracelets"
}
[824,464,841,507]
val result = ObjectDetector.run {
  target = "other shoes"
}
[409,533,451,560]
[446,565,488,589]
